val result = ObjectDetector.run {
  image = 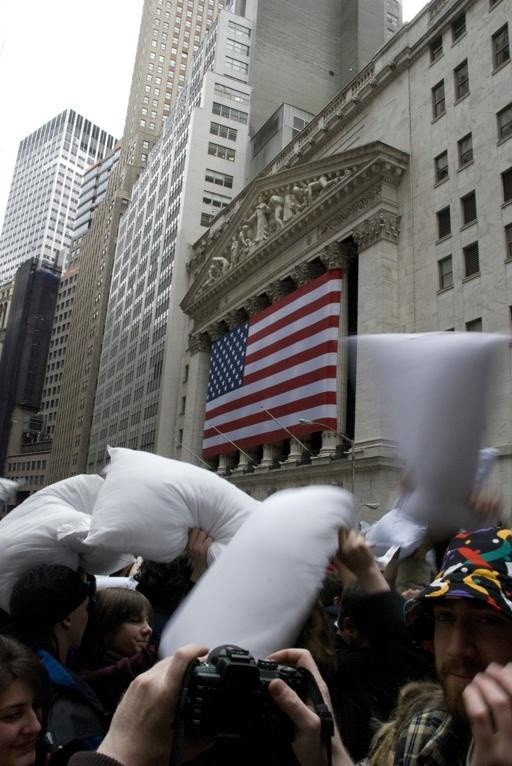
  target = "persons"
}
[198,169,364,290]
[1,525,512,766]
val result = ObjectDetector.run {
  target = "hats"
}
[9,563,90,641]
[402,525,512,657]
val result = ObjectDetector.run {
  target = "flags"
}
[201,266,344,463]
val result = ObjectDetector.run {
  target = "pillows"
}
[86,446,258,578]
[157,488,358,658]
[2,473,137,615]
[357,333,509,548]
[366,446,497,560]
[1,478,18,501]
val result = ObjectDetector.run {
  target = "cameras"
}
[178,644,305,753]
